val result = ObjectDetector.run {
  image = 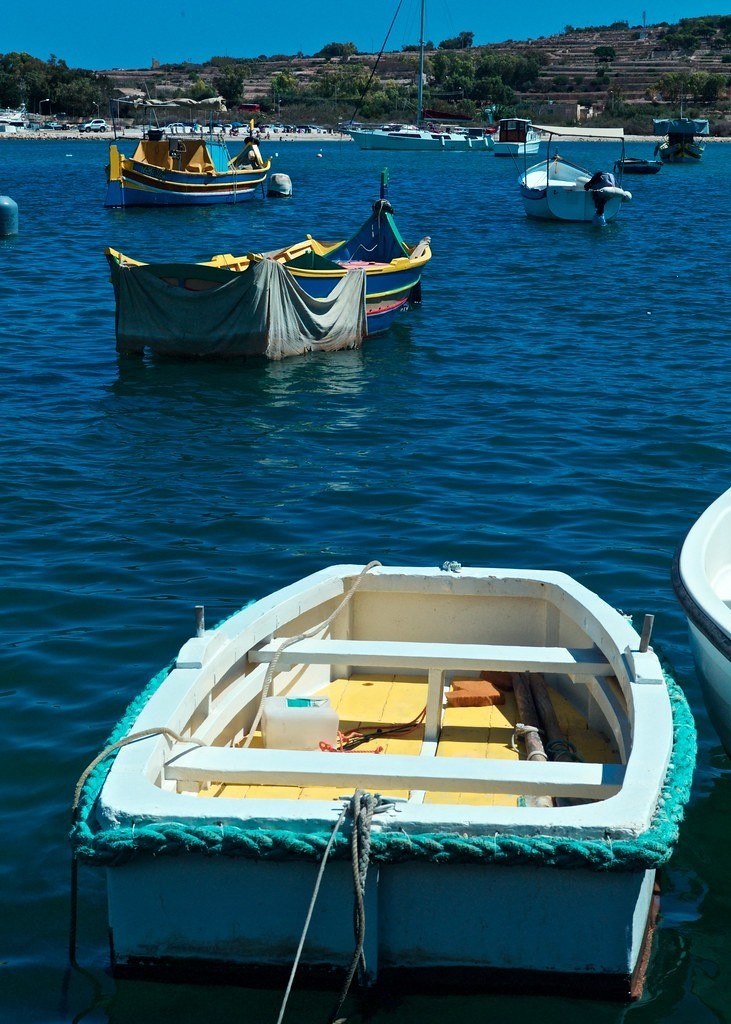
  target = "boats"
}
[494,118,542,155]
[517,123,632,224]
[103,165,433,363]
[160,123,283,136]
[104,97,271,205]
[615,158,663,174]
[70,562,698,1024]
[0,100,31,133]
[651,94,709,164]
[671,484,731,722]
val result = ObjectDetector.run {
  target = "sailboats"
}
[335,0,498,151]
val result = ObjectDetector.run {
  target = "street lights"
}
[38,99,49,114]
[93,102,99,119]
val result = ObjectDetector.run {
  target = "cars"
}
[280,124,293,133]
[299,125,319,131]
[78,119,108,133]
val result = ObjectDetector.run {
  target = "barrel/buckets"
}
[261,697,338,751]
